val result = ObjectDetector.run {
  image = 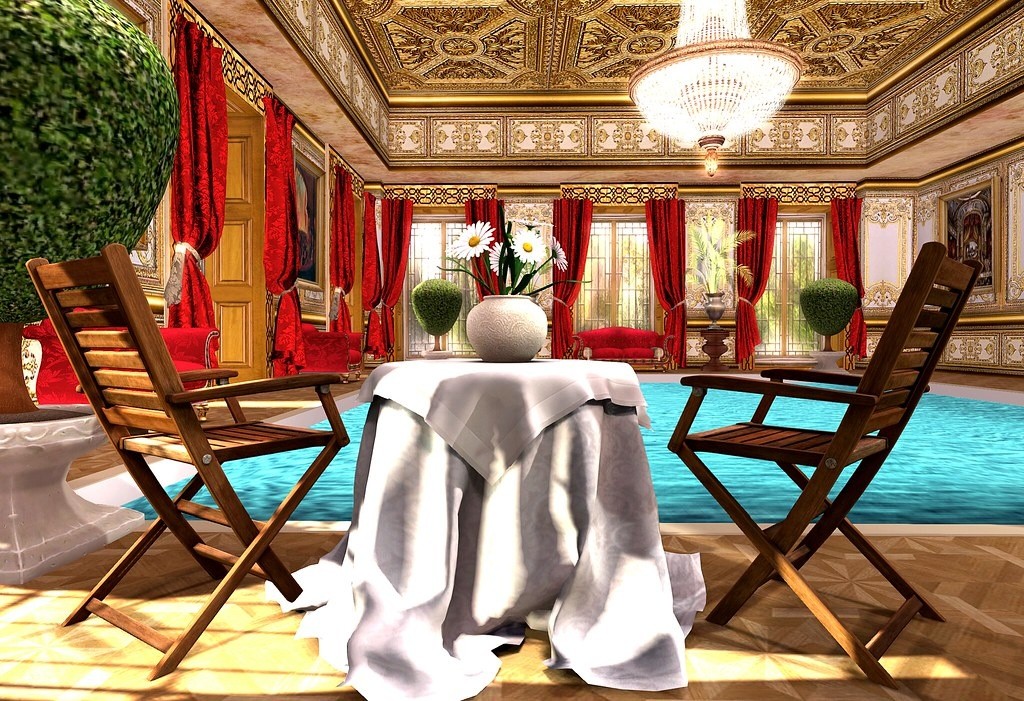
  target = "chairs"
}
[24,238,354,685]
[667,241,985,690]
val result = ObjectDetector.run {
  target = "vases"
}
[465,294,548,362]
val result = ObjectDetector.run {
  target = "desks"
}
[263,361,708,700]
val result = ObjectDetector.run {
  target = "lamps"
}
[627,0,803,177]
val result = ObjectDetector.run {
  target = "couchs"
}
[23,307,220,404]
[299,323,365,383]
[571,327,675,373]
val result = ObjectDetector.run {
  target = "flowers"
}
[436,216,593,295]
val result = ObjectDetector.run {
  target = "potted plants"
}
[0,0,178,588]
[411,278,462,359]
[798,277,858,374]
[669,212,755,331]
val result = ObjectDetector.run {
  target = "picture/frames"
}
[935,174,1004,295]
[291,144,326,292]
